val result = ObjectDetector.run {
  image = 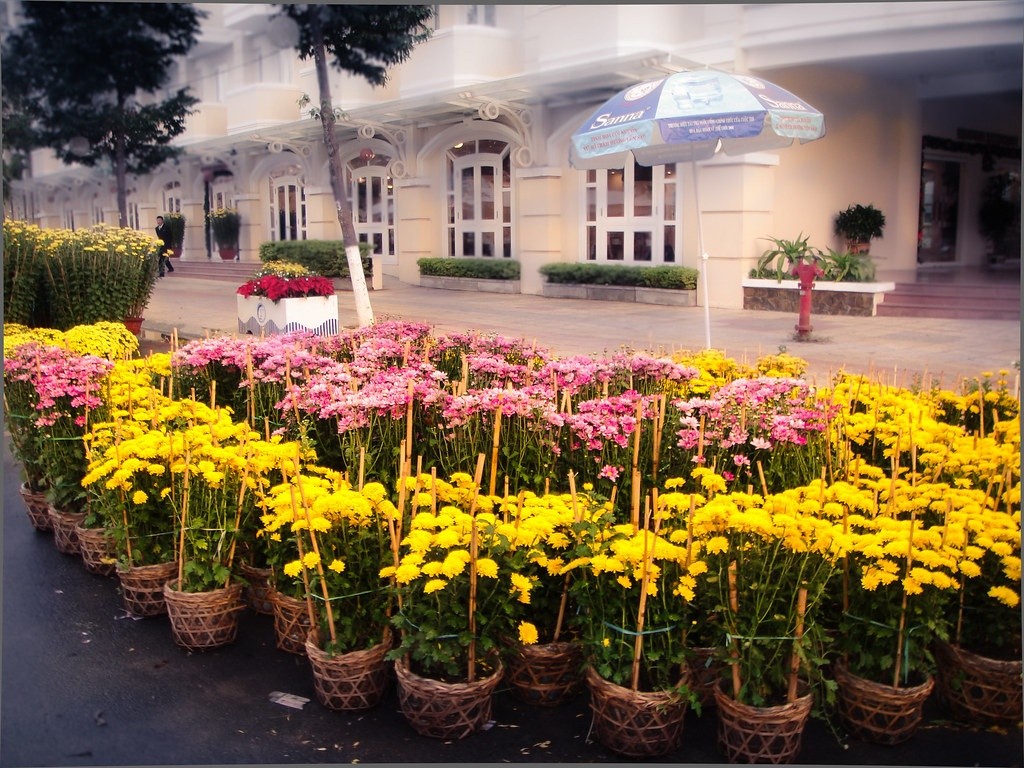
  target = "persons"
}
[154,215,175,278]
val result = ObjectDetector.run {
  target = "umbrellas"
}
[568,69,826,350]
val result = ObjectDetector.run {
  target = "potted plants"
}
[417,256,456,288]
[476,259,521,294]
[635,266,700,307]
[584,264,642,302]
[834,203,887,255]
[977,198,1018,264]
[538,262,599,299]
[444,258,484,291]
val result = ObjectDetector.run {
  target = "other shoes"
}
[158,274,165,277]
[168,268,172,272]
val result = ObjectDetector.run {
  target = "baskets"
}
[18,482,1024,766]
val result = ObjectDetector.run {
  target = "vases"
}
[584,651,691,759]
[74,519,136,577]
[832,664,936,744]
[305,627,393,713]
[239,555,279,616]
[266,577,335,657]
[19,481,55,532]
[218,248,237,259]
[114,561,183,617]
[500,627,584,708]
[169,248,182,258]
[941,641,1023,726]
[393,645,504,744]
[714,677,814,765]
[237,293,338,336]
[163,577,246,651]
[47,501,89,553]
[686,644,728,710]
[122,317,145,335]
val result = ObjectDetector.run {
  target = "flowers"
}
[4,205,1021,717]
